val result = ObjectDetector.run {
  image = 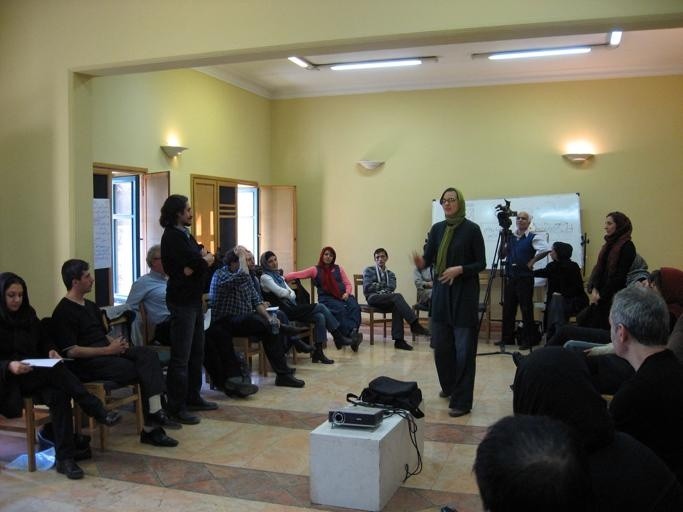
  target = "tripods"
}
[476,230,535,356]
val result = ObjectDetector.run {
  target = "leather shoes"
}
[411,320,428,334]
[440,390,469,416]
[187,398,218,410]
[395,339,412,349]
[224,377,258,398]
[95,406,122,426]
[281,323,311,337]
[495,340,537,350]
[141,410,181,446]
[276,368,305,387]
[167,408,199,424]
[55,457,83,479]
[295,340,313,353]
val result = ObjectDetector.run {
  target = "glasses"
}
[441,198,457,203]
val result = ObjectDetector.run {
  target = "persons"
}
[532,242,590,347]
[362,248,428,351]
[208,246,310,387]
[284,246,363,352]
[629,253,649,271]
[51,258,183,448]
[414,267,433,308]
[159,195,219,425]
[258,252,354,364]
[587,212,636,315]
[472,268,682,511]
[493,209,552,350]
[413,186,487,416]
[1,272,123,481]
[125,243,259,400]
[246,250,314,376]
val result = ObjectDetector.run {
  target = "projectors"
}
[328,406,384,428]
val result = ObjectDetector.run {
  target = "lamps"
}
[358,160,384,172]
[470,27,623,65]
[159,140,188,160]
[557,148,596,165]
[286,50,439,75]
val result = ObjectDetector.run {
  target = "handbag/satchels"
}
[38,422,92,461]
[362,377,422,410]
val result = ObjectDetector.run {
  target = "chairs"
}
[354,273,394,346]
[0,279,317,473]
[412,302,489,343]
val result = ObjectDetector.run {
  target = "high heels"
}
[312,347,333,363]
[334,334,362,352]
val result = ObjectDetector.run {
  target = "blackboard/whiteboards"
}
[432,192,584,270]
